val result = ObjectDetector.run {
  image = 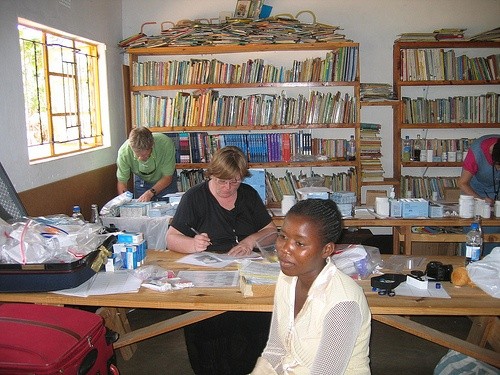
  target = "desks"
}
[0,202,499,369]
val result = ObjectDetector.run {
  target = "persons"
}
[117,127,178,203]
[459,134,500,259]
[249,199,371,375]
[164,146,280,375]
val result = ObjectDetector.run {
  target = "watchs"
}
[150,187,156,196]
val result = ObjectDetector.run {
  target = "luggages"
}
[0,302,120,375]
[0,163,125,293]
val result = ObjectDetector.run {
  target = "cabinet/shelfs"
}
[123,41,500,185]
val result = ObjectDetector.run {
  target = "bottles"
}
[403,136,411,161]
[494,201,500,217]
[414,134,422,162]
[389,187,395,199]
[89,204,99,224]
[465,223,481,271]
[72,206,85,224]
[472,215,484,260]
[349,135,356,161]
[177,179,181,192]
[281,195,297,215]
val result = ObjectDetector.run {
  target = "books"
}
[131,89,357,128]
[401,91,500,125]
[401,134,476,163]
[164,133,354,164]
[116,0,354,48]
[176,167,358,203]
[360,82,393,102]
[360,122,386,183]
[394,25,500,42]
[400,175,461,198]
[99,191,373,219]
[290,45,359,82]
[400,48,500,81]
[132,58,292,86]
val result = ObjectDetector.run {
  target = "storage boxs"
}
[390,198,429,217]
[113,232,146,269]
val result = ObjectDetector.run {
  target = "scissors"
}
[378,288,395,297]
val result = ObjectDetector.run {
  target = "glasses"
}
[218,180,239,186]
[138,160,156,175]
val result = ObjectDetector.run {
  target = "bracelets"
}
[192,239,195,253]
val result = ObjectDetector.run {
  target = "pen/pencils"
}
[191,227,212,245]
[484,190,489,198]
